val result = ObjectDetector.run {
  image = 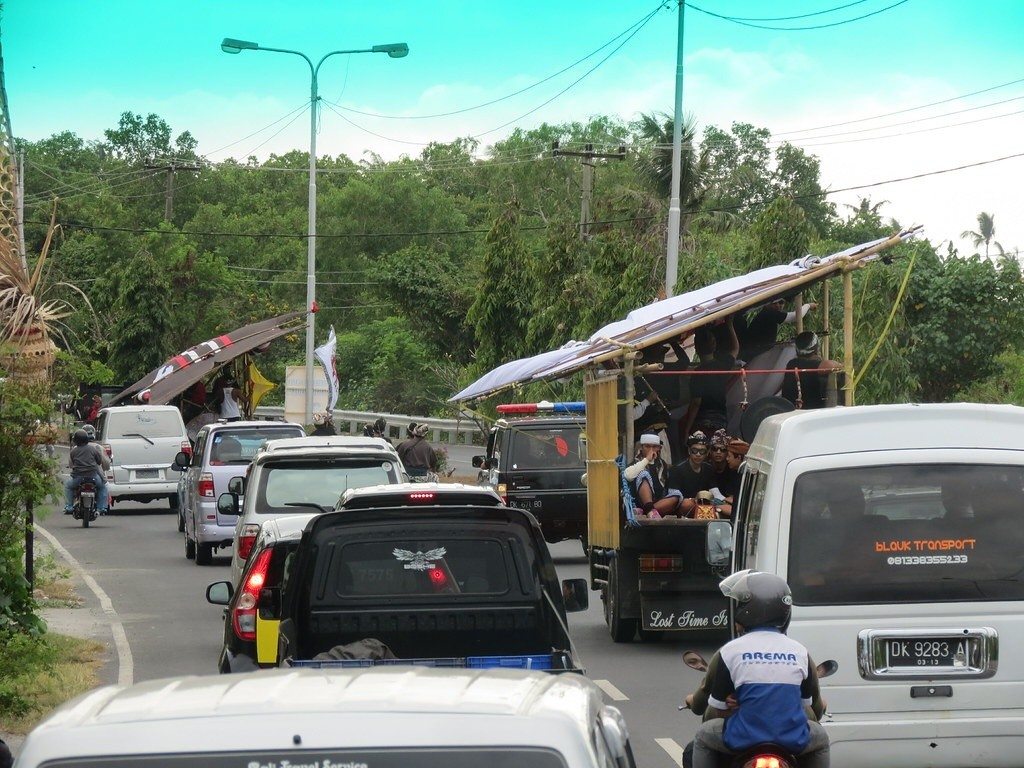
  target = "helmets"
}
[733,572,792,634]
[312,409,332,425]
[374,418,386,433]
[363,425,373,436]
[73,430,89,445]
[82,425,96,442]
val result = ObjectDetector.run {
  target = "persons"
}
[63,424,111,514]
[683,569,831,768]
[395,423,440,483]
[309,411,337,435]
[622,299,843,519]
[562,581,580,610]
[685,653,705,671]
[481,458,498,470]
[183,365,254,425]
[363,416,392,444]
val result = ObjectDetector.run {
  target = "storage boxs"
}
[289,654,553,672]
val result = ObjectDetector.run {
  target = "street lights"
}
[220,38,409,437]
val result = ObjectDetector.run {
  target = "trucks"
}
[582,261,866,644]
[258,504,589,678]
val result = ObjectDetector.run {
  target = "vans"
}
[90,405,191,508]
[175,419,309,566]
[704,402,1024,768]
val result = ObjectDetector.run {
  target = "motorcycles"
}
[65,455,114,528]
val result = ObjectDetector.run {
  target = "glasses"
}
[773,300,784,304]
[711,447,727,453]
[690,448,708,455]
[643,444,658,450]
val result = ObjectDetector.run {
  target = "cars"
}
[206,483,508,675]
[218,435,412,592]
[170,454,192,531]
[471,413,589,558]
[821,484,945,520]
[13,666,639,768]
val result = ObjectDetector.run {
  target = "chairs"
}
[218,437,242,461]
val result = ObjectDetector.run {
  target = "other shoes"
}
[65,505,73,515]
[647,508,662,519]
[96,508,107,513]
[632,507,644,516]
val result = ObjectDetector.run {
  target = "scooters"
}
[678,650,839,768]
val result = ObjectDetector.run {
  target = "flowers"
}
[433,445,448,469]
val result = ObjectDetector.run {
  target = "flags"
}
[313,324,339,413]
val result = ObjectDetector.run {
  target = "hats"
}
[695,330,717,352]
[686,431,709,447]
[770,295,785,305]
[645,343,670,354]
[407,423,417,438]
[640,434,660,445]
[728,440,749,455]
[711,428,733,449]
[225,378,236,384]
[413,423,429,437]
